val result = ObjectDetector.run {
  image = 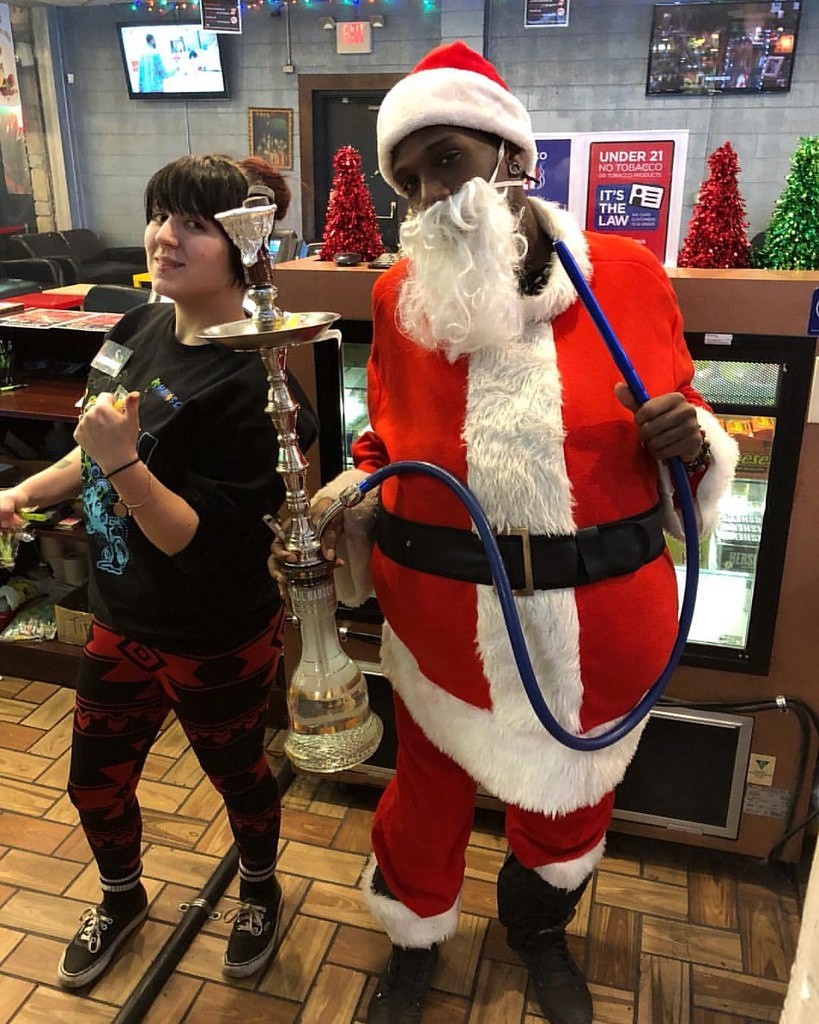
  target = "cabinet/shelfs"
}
[0,299,130,660]
[266,253,819,870]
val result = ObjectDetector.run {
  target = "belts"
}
[372,483,667,587]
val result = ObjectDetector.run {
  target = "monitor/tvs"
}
[644,0,802,96]
[116,18,229,100]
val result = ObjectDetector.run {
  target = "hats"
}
[375,40,537,182]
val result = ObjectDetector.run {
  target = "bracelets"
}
[119,472,154,517]
[99,458,144,480]
[684,429,712,479]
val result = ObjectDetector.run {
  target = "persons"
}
[239,156,322,457]
[308,40,740,1023]
[187,48,203,73]
[137,35,175,94]
[0,152,323,992]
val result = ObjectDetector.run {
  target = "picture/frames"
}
[247,105,294,173]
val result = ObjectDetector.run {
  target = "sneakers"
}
[223,878,283,978]
[508,923,593,1024]
[56,894,149,988]
[366,939,439,1024]
